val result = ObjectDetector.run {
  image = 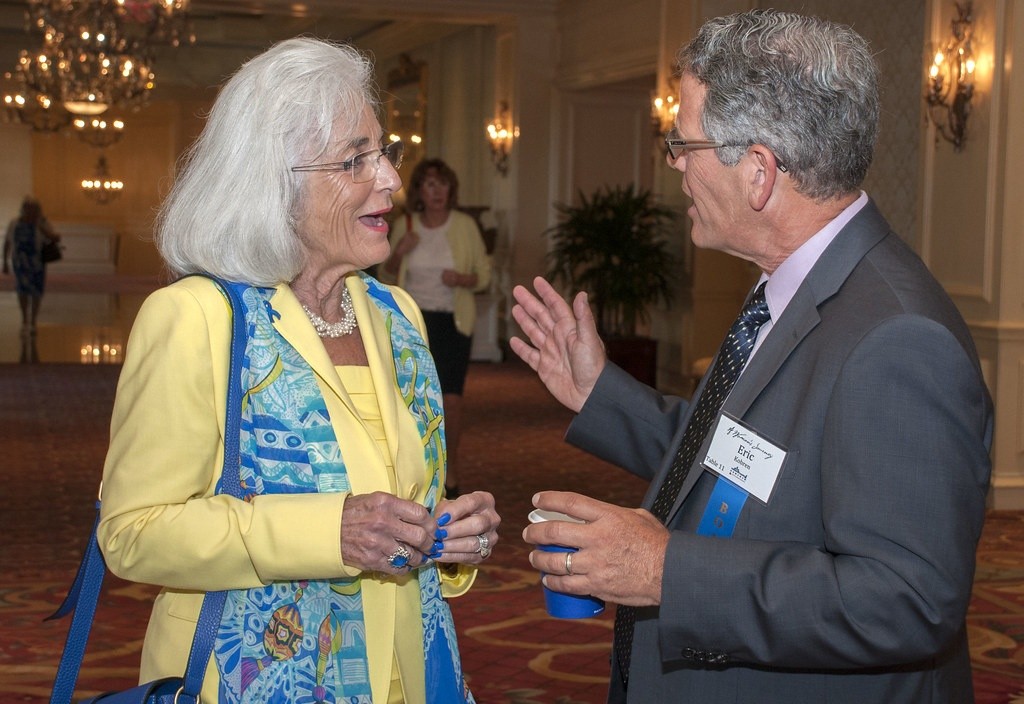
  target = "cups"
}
[528,508,606,619]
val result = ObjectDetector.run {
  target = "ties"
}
[607,278,771,704]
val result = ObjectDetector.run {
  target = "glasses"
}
[664,126,789,174]
[291,140,404,184]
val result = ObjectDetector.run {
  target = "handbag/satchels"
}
[80,676,198,704]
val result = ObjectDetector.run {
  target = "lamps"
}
[923,0,978,150]
[649,96,679,156]
[0,0,199,206]
[481,119,509,177]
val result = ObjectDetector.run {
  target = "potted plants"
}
[541,182,687,389]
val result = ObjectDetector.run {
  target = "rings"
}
[474,533,489,557]
[388,540,412,568]
[566,551,573,575]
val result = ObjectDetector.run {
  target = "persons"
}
[94,35,501,704]
[2,196,62,362]
[379,158,491,499]
[509,8,995,704]
